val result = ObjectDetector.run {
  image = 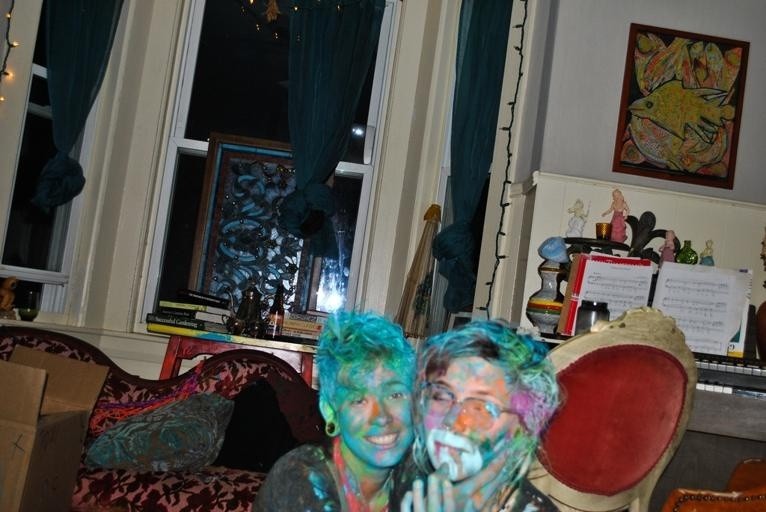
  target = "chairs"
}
[658,460,765,511]
[506,306,697,512]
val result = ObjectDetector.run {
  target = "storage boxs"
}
[1,343,107,511]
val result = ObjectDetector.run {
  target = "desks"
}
[145,313,319,380]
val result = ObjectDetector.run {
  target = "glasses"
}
[421,385,524,428]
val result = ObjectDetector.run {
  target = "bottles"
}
[264,283,286,339]
[676,241,698,265]
[575,300,610,333]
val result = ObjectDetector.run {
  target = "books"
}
[145,289,232,330]
[557,248,754,358]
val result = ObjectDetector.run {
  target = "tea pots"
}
[225,279,273,320]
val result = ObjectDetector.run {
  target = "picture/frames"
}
[612,22,751,191]
[186,132,335,314]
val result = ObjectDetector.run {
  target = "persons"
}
[253,311,418,512]
[388,311,558,512]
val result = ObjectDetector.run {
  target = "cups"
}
[19,290,41,322]
[226,317,264,337]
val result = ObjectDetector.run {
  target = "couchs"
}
[0,323,333,511]
